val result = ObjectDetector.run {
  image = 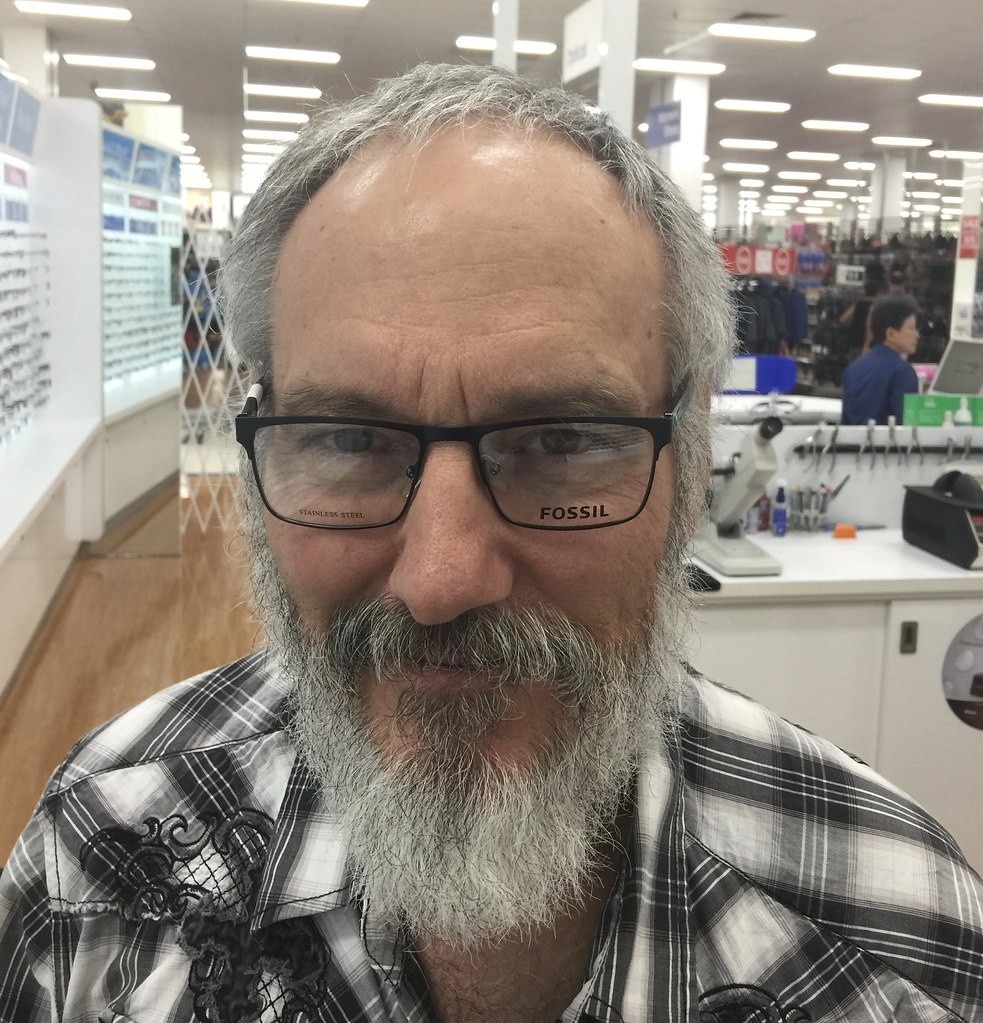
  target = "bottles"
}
[954,398,972,424]
[940,410,954,427]
[772,488,787,537]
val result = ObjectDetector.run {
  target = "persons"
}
[0,62,983,1022]
[842,289,919,427]
[840,281,882,367]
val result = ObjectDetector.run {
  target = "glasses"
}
[233,372,695,532]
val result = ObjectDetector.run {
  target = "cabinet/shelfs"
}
[711,394,842,424]
[670,528,983,878]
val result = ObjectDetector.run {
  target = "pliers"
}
[786,420,841,474]
[884,414,902,468]
[905,422,925,467]
[856,418,877,469]
